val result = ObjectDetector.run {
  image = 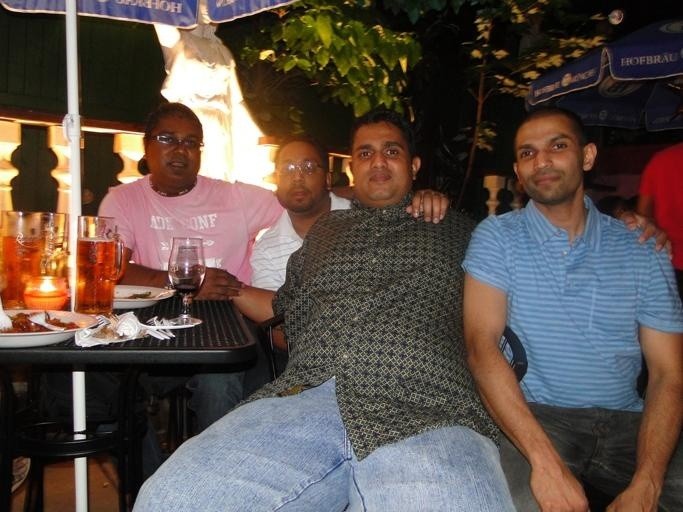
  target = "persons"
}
[91,102,454,481]
[596,194,631,220]
[248,133,361,295]
[457,105,682,512]
[634,140,682,303]
[125,108,676,512]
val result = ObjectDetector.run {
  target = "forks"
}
[26,311,64,331]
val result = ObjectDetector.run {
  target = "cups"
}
[74,215,121,317]
[0,210,71,310]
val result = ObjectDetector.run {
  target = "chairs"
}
[258,313,285,381]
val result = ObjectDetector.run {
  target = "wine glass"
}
[167,236,206,328]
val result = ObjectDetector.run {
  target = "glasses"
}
[147,134,206,149]
[279,159,327,176]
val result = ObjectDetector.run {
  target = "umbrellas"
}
[0,1,292,512]
[523,22,683,134]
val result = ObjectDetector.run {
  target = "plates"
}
[112,284,175,310]
[0,308,100,350]
